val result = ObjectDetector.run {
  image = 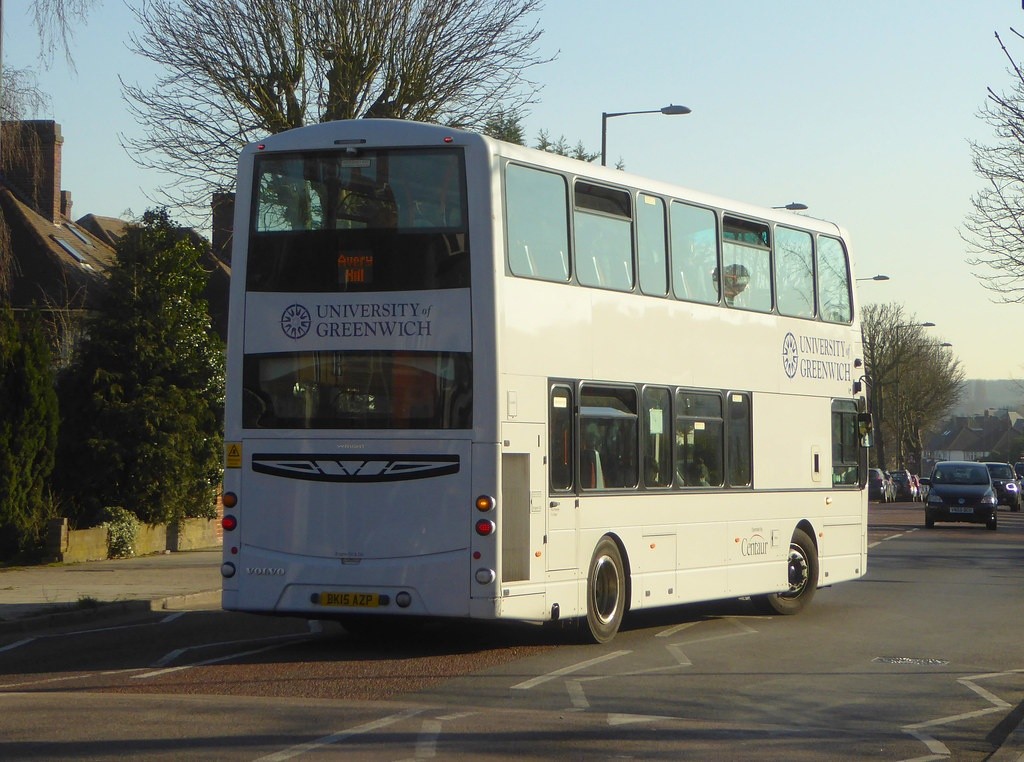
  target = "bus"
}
[222,116,874,644]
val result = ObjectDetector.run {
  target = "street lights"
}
[895,323,936,471]
[598,101,693,170]
[915,342,952,474]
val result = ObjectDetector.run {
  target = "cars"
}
[884,471,897,502]
[919,461,997,530]
[869,467,892,503]
[920,477,931,503]
[1013,461,1024,500]
[984,462,1023,513]
[911,474,923,502]
[890,469,917,502]
